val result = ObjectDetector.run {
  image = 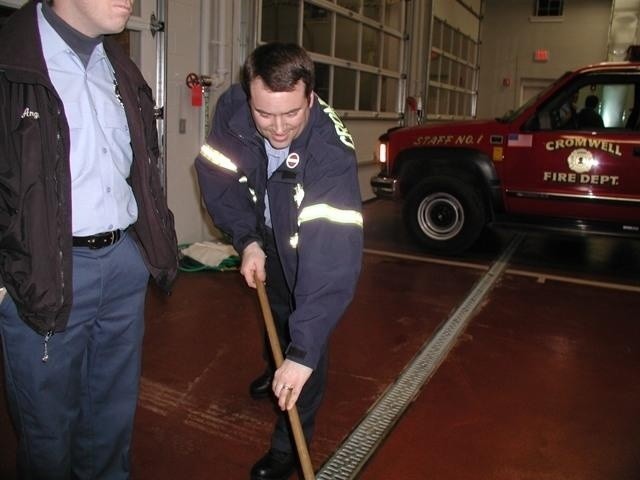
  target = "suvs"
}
[369,58,640,267]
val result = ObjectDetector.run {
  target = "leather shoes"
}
[249,447,300,479]
[249,367,279,400]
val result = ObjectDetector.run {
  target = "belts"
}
[70,228,122,252]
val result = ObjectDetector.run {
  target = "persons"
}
[192,38,365,479]
[1,0,184,478]
[556,89,604,132]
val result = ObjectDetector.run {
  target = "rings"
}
[283,386,293,391]
